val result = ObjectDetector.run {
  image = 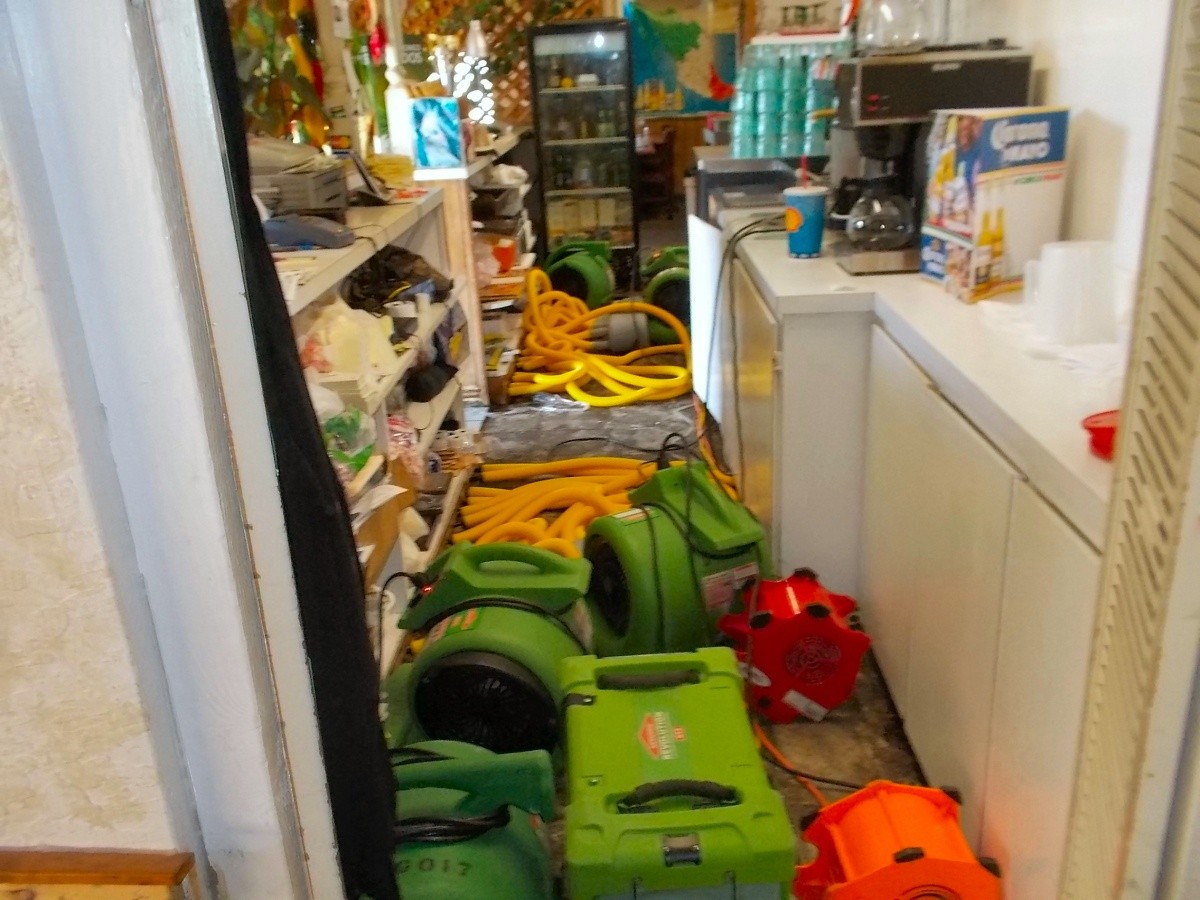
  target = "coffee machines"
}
[831,39,1033,280]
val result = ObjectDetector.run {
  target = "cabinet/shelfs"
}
[683,175,871,604]
[413,125,539,403]
[861,322,1024,862]
[271,187,490,680]
[980,477,1103,899]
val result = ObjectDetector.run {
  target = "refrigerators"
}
[529,18,642,297]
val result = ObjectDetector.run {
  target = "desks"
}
[641,115,707,198]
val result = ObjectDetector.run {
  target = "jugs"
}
[846,175,916,248]
[843,0,931,56]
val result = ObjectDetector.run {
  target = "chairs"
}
[639,130,679,219]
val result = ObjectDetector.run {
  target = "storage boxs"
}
[559,647,798,900]
[482,312,527,405]
[475,231,517,273]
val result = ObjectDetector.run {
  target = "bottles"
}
[926,113,1006,292]
[535,53,632,191]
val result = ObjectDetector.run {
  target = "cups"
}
[783,186,831,258]
[728,39,831,157]
